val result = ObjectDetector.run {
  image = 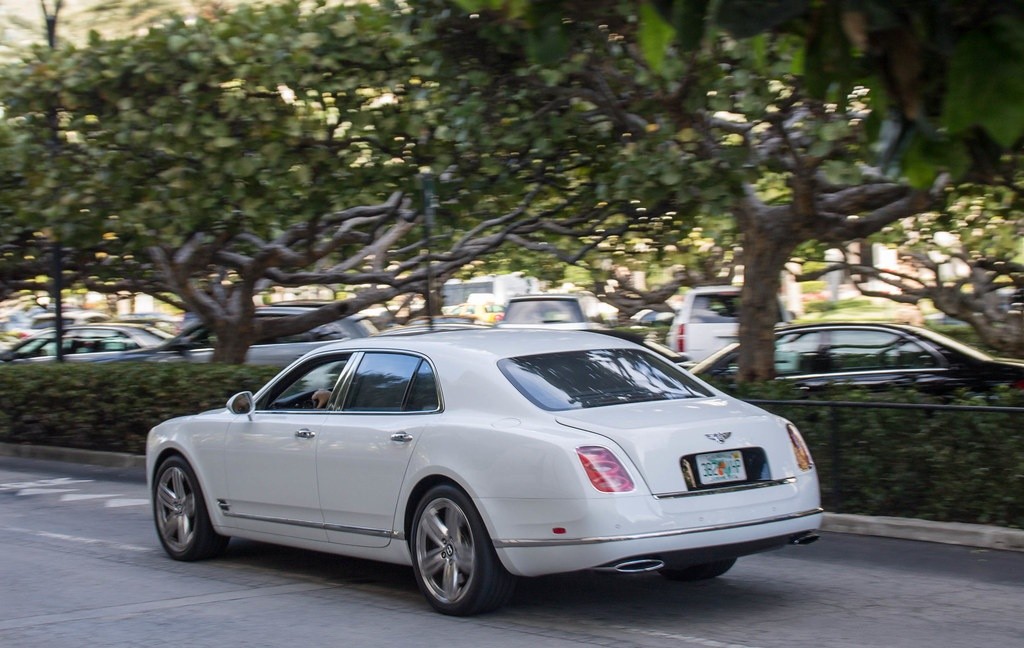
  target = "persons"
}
[236,397,251,413]
[312,388,331,409]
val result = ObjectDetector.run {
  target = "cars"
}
[498,294,593,331]
[143,332,825,617]
[667,286,796,365]
[684,320,1024,394]
[0,301,379,364]
